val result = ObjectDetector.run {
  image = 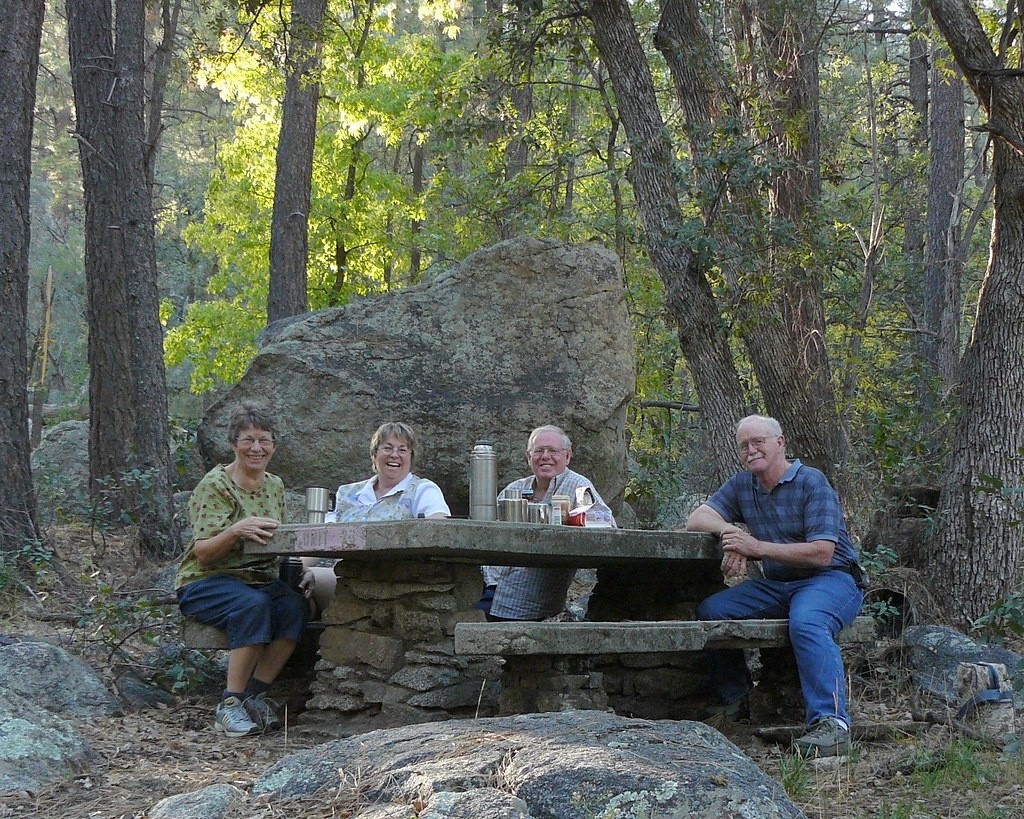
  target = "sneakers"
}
[793,716,849,756]
[216,692,282,738]
[706,699,748,721]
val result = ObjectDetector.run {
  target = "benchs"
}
[184,619,324,713]
[454,617,872,729]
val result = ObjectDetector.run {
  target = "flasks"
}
[469,441,498,521]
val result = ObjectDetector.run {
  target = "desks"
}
[243,518,763,742]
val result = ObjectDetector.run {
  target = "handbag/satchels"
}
[948,661,1015,741]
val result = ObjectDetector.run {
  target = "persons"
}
[478,426,620,625]
[174,400,314,739]
[298,421,451,623]
[686,414,869,756]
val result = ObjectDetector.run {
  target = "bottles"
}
[551,496,570,525]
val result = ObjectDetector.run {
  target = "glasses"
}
[531,448,567,456]
[377,445,412,457]
[739,435,777,452]
[236,437,275,446]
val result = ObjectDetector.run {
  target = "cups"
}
[305,486,336,523]
[528,499,550,524]
[498,490,528,522]
